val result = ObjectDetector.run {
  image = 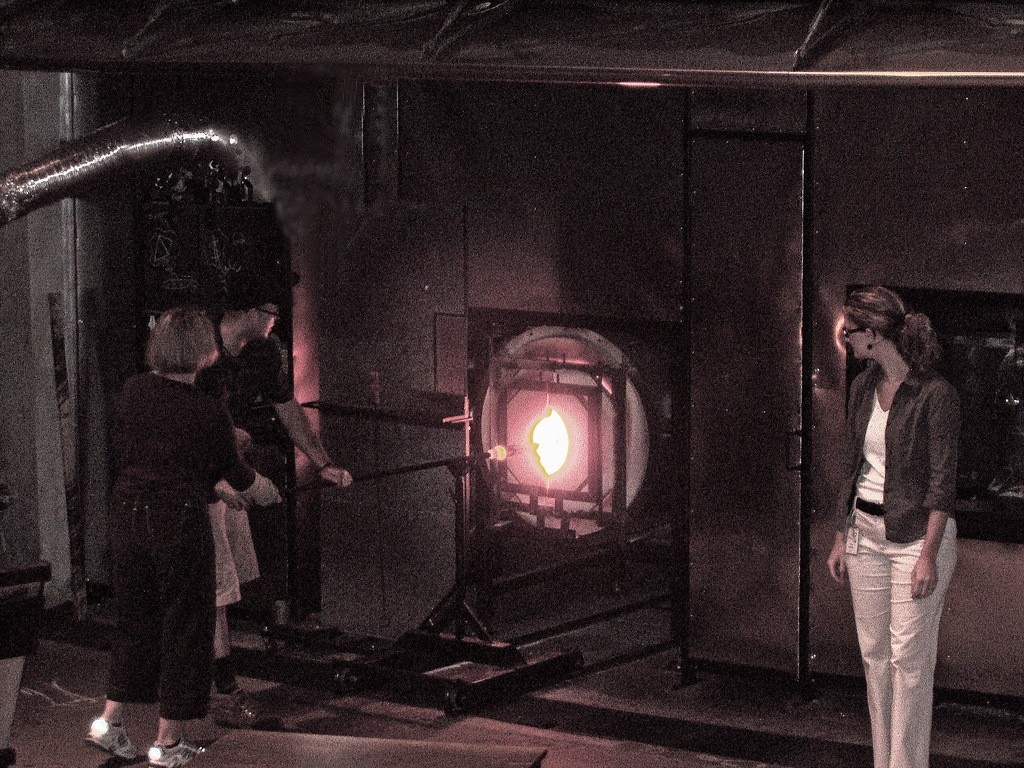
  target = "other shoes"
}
[210,679,259,714]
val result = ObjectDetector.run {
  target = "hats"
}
[219,245,301,310]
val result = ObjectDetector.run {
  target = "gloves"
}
[241,468,283,508]
[213,479,249,511]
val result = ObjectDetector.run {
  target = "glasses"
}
[245,306,283,324]
[842,325,876,339]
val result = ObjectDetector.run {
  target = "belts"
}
[851,498,886,516]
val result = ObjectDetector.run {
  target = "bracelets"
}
[314,458,334,475]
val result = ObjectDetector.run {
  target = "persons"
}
[826,282,962,768]
[81,239,359,765]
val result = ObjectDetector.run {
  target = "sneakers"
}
[148,733,206,768]
[85,717,137,760]
